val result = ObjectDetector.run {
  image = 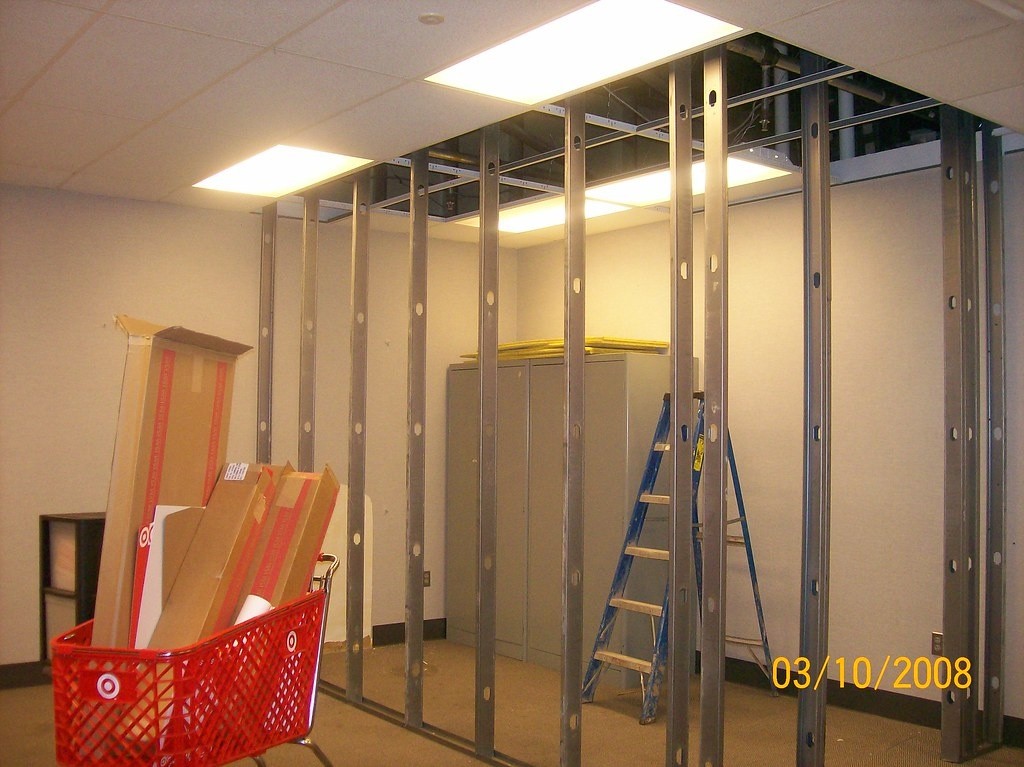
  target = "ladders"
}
[576,390,781,727]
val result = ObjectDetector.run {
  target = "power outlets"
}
[932,631,944,657]
[424,570,430,587]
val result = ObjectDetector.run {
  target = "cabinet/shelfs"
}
[448,352,701,694]
[40,513,106,677]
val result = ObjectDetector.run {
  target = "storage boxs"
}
[91,313,340,745]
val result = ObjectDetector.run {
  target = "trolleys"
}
[48,553,340,767]
